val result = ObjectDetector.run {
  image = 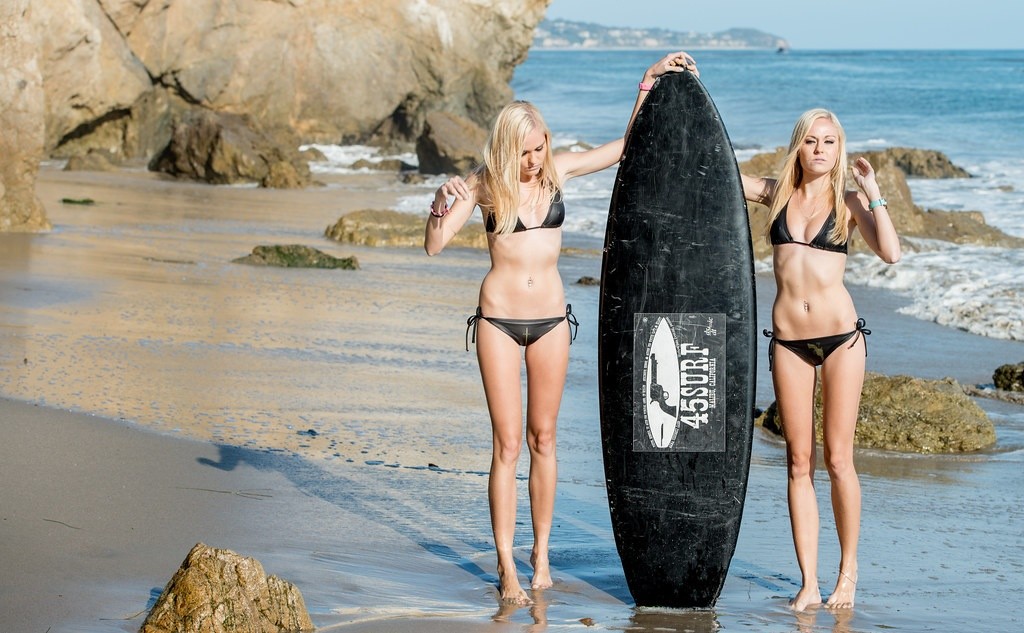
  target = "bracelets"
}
[430,200,449,218]
[639,82,651,90]
[868,198,888,212]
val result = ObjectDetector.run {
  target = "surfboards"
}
[596,65,758,613]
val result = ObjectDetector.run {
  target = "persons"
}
[672,52,900,611]
[424,53,698,606]
